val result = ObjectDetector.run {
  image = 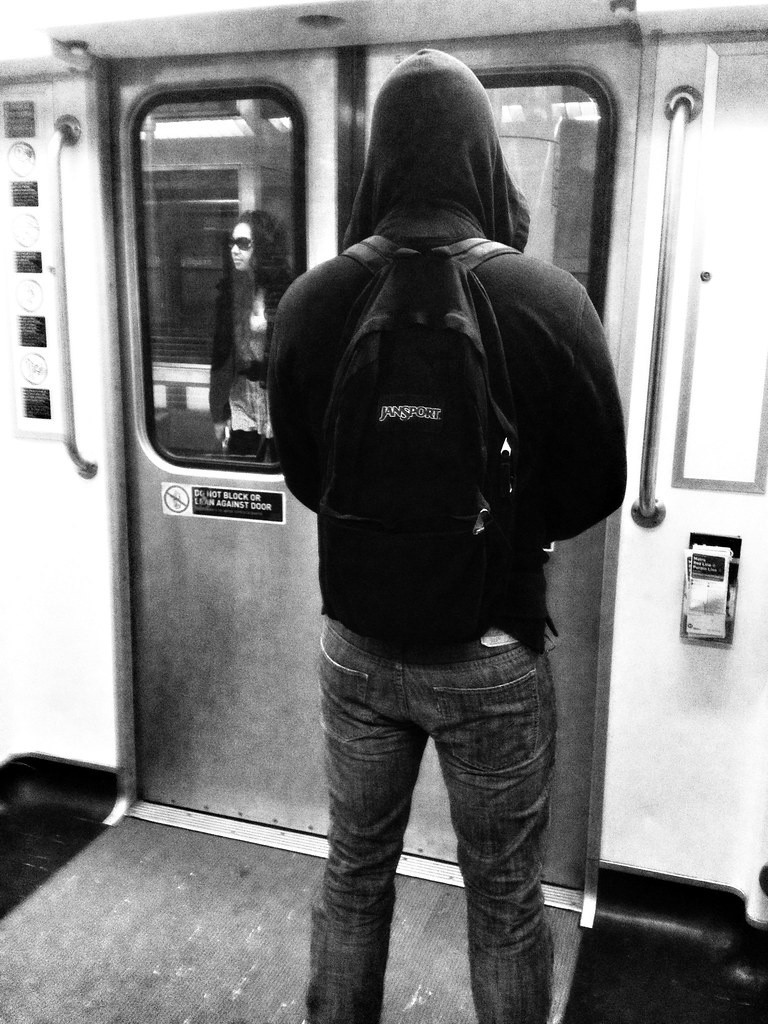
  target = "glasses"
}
[227,235,253,252]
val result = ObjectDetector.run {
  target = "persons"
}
[264,53,626,1024]
[209,212,290,472]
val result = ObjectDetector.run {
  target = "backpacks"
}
[315,233,518,550]
